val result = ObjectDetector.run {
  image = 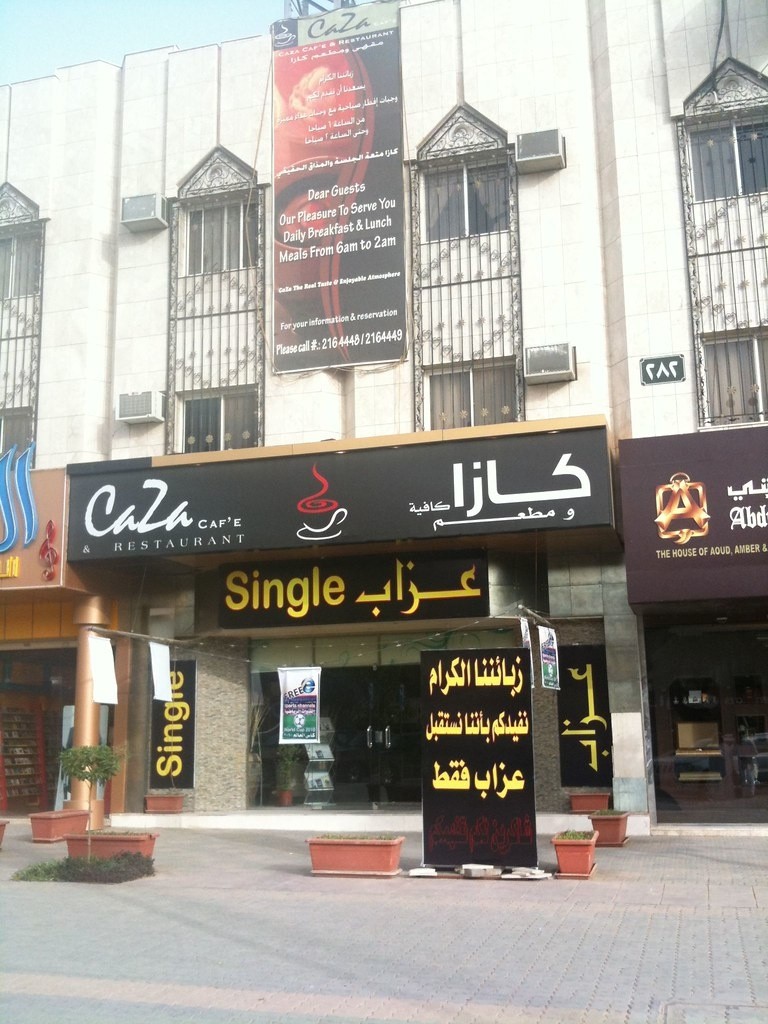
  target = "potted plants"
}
[587,810,630,847]
[144,791,186,814]
[551,829,600,879]
[275,742,298,805]
[64,830,160,860]
[305,833,406,873]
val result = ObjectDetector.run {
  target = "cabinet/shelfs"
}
[1,694,60,808]
[299,717,339,807]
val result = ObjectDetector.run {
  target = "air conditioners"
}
[115,390,167,424]
[522,343,576,384]
[121,193,169,234]
[514,128,565,175]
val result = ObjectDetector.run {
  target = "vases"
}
[0,820,11,845]
[29,810,95,843]
[567,794,611,812]
